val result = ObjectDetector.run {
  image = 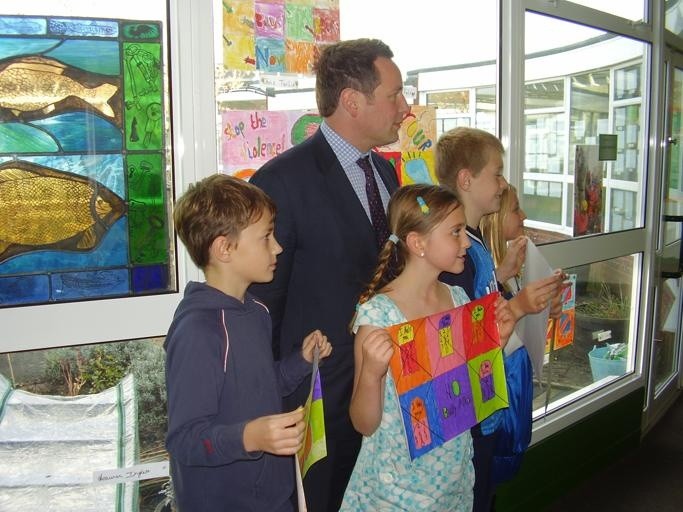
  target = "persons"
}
[248,39,411,512]
[482,187,573,322]
[162,173,333,512]
[434,127,561,511]
[340,183,514,512]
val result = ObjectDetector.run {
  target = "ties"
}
[356,156,396,278]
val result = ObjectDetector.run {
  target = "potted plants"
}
[573,280,630,361]
